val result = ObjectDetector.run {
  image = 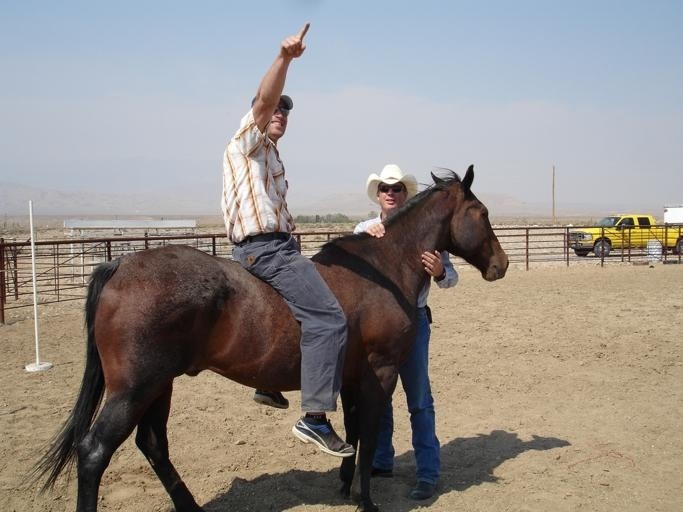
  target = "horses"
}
[25,162,512,512]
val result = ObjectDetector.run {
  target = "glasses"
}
[274,106,289,117]
[378,186,405,193]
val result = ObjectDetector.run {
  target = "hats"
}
[251,95,293,110]
[365,164,418,206]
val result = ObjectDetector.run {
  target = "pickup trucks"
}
[566,214,683,257]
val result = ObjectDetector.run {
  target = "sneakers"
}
[253,388,289,409]
[367,468,393,478]
[291,416,356,458]
[409,480,438,500]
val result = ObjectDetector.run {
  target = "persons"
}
[351,162,459,500]
[219,21,356,458]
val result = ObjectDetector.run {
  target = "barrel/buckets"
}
[648,239,662,263]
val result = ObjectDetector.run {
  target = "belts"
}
[233,231,289,247]
